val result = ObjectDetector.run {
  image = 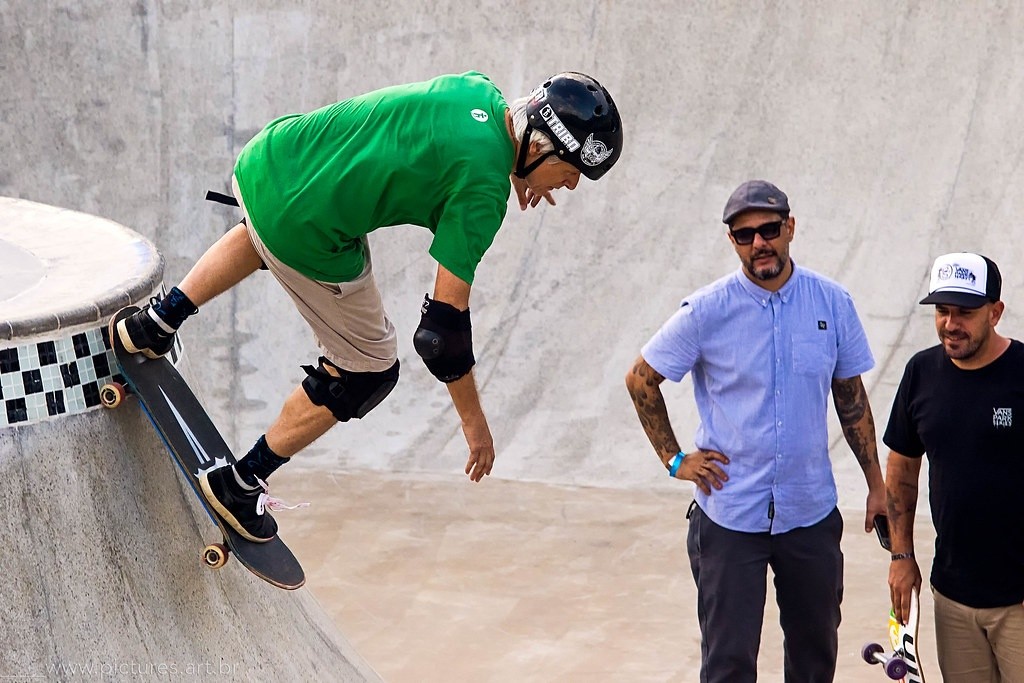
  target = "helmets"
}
[526,71,623,181]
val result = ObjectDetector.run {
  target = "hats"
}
[722,180,790,226]
[918,253,1002,308]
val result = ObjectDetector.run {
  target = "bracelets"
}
[891,552,915,561]
[665,455,676,470]
[670,452,685,478]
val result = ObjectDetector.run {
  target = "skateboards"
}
[98,304,307,593]
[859,584,926,683]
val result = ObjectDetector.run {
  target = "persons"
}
[116,68,623,542]
[626,180,888,683]
[882,250,1024,683]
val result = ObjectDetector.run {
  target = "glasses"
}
[730,220,786,246]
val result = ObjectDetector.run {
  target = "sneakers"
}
[117,296,175,359]
[199,466,309,543]
